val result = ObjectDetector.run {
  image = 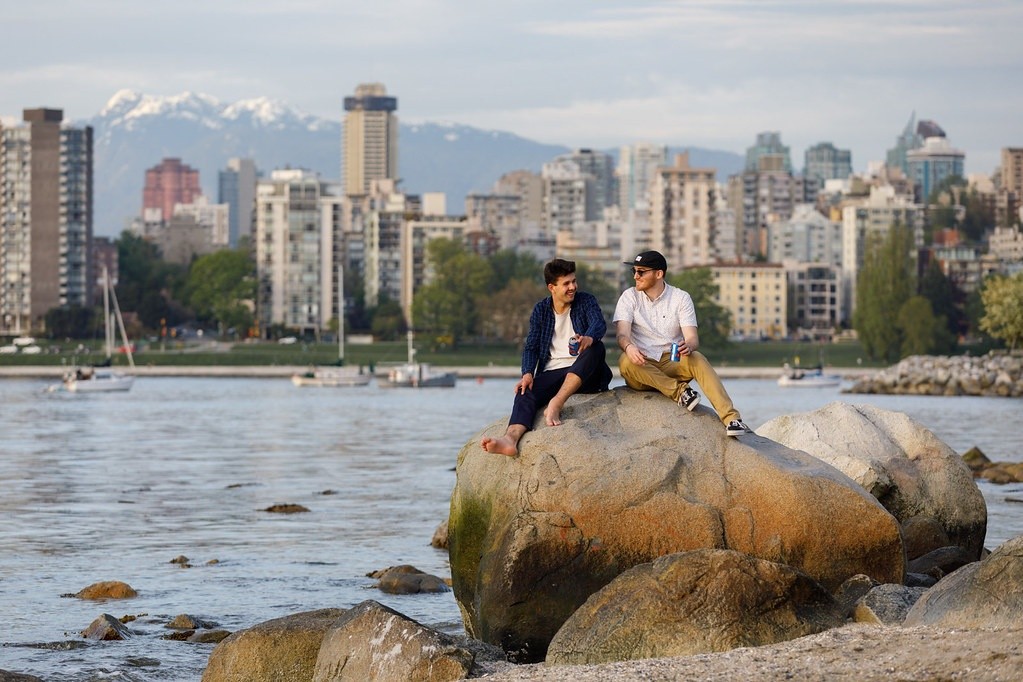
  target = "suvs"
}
[74,343,91,354]
[82,341,83,345]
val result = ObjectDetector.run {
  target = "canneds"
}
[669,342,680,362]
[568,337,579,356]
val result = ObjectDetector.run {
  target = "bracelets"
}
[625,343,634,353]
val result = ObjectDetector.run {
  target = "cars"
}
[0,345,19,354]
[278,335,298,345]
[118,344,137,353]
[22,344,42,354]
[45,345,64,354]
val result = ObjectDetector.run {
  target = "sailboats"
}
[374,331,459,387]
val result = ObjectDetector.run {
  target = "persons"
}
[613,251,746,436]
[76,367,89,380]
[482,259,613,456]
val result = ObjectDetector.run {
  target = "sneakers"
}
[726,419,748,436]
[677,387,702,412]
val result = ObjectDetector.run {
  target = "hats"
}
[623,251,667,271]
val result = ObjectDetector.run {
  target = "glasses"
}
[631,267,650,277]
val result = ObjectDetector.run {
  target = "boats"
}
[291,372,373,386]
[778,372,855,387]
[50,365,135,392]
